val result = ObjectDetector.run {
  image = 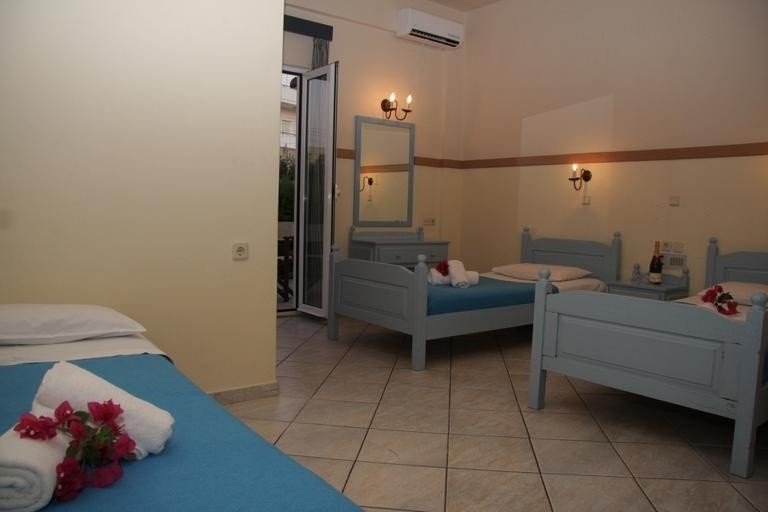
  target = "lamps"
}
[360,176,373,191]
[568,165,591,190]
[380,93,413,121]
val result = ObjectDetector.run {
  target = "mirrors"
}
[353,116,414,228]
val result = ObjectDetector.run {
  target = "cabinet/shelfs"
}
[349,242,448,275]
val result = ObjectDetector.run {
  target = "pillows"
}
[698,281,768,305]
[492,263,592,282]
[0,303,147,345]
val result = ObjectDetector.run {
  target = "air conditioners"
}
[396,9,463,50]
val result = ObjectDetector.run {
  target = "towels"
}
[30,361,175,461]
[447,260,469,288]
[428,268,479,286]
[0,422,83,512]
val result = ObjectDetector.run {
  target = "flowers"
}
[13,399,136,502]
[436,260,449,276]
[701,284,737,314]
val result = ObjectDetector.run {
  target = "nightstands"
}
[605,265,689,301]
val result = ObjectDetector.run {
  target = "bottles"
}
[649,240,662,284]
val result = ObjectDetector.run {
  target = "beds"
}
[528,238,767,480]
[328,227,622,376]
[1,333,366,512]
